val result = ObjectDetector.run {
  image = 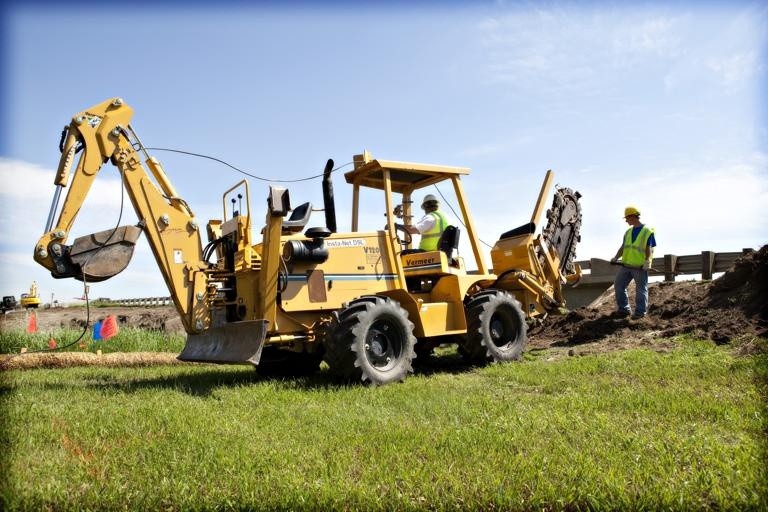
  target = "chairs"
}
[401,225,460,265]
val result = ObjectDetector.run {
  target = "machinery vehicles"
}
[0,278,41,314]
[27,90,590,386]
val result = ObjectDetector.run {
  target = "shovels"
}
[610,261,665,273]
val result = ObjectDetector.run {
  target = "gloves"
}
[644,260,651,271]
[611,257,617,263]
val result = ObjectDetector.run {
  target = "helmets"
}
[624,207,640,219]
[421,195,439,209]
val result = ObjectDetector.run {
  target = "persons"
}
[607,205,658,321]
[397,195,451,298]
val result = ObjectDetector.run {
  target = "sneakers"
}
[610,309,643,319]
